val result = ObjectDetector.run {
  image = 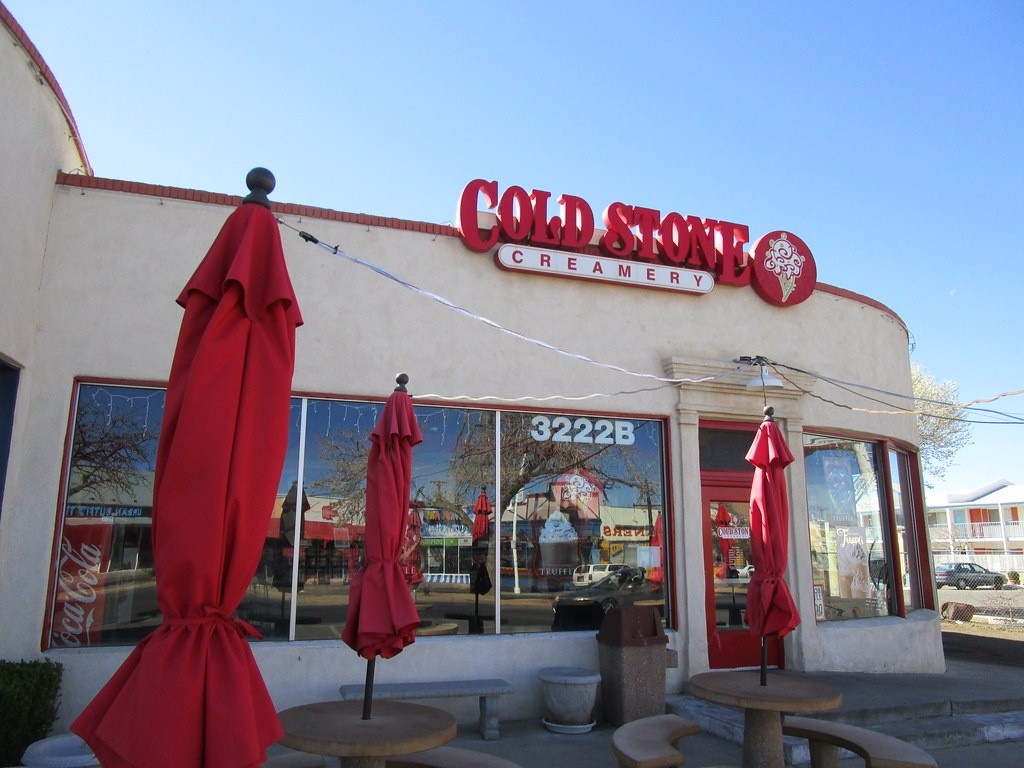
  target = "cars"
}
[934,562,1008,591]
[552,566,665,622]
[573,562,630,586]
[716,564,755,578]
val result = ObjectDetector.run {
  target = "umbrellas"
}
[69,166,305,768]
[647,510,666,593]
[715,502,737,562]
[469,485,493,615]
[341,374,424,720]
[399,500,423,592]
[272,481,310,620]
[745,408,801,687]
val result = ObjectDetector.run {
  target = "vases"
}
[538,667,603,735]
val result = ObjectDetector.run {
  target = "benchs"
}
[386,746,524,768]
[338,677,517,741]
[260,750,327,768]
[612,713,701,768]
[782,716,938,768]
[414,622,459,636]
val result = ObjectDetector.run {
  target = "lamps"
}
[746,365,784,392]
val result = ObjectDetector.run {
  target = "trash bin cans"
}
[551,599,604,631]
[596,605,669,728]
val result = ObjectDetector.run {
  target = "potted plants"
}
[1007,571,1020,586]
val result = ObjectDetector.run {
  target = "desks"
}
[248,612,322,640]
[276,699,458,768]
[415,604,434,611]
[690,671,842,768]
[444,612,496,634]
